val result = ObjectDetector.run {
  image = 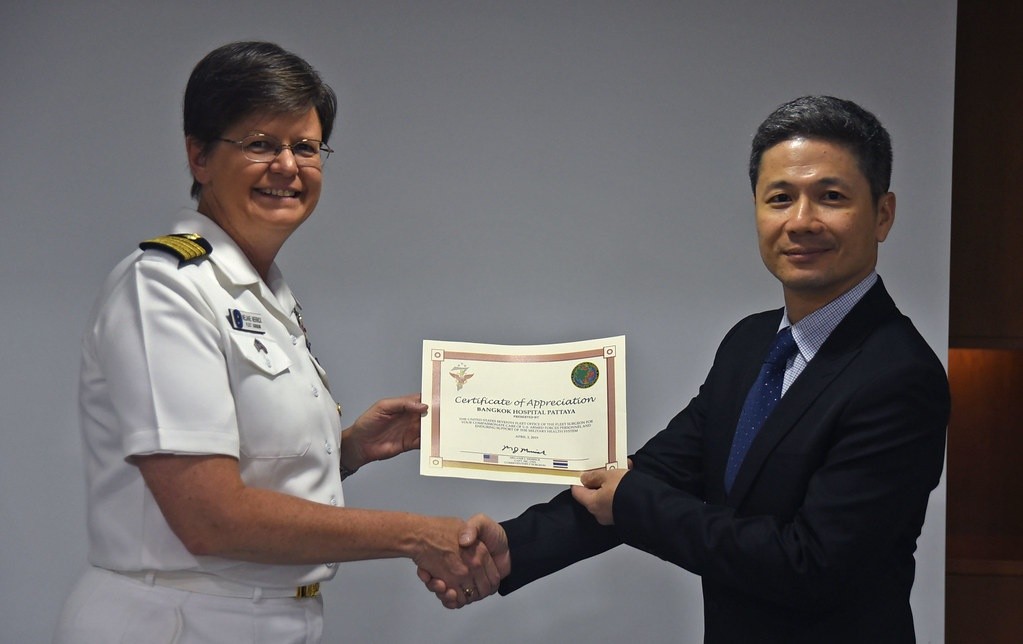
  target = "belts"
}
[119,570,318,599]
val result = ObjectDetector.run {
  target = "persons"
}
[417,94,951,644]
[53,40,501,644]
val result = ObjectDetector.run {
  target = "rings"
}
[460,582,474,598]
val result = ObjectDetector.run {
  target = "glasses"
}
[203,133,335,171]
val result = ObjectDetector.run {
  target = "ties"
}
[725,326,800,500]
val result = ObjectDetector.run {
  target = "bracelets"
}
[340,459,358,483]
